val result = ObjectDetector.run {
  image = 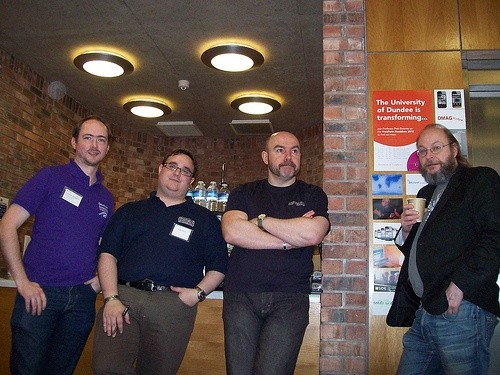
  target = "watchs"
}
[257,213,267,229]
[195,286,206,302]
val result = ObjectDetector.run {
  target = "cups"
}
[406,197,426,222]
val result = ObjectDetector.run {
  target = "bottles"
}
[205,181,220,211]
[194,180,207,209]
[186,184,194,202]
[218,184,231,211]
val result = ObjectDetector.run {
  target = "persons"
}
[0,116,116,375]
[373,199,403,219]
[92,149,228,375]
[222,131,332,375]
[387,124,500,375]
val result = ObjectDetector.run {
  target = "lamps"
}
[123,101,172,118]
[201,46,264,72]
[231,97,281,115]
[74,53,134,78]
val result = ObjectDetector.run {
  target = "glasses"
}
[417,142,453,157]
[164,163,193,177]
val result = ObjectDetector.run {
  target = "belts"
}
[119,281,175,292]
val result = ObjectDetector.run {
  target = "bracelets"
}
[283,241,286,250]
[104,294,119,305]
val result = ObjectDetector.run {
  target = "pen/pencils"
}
[111,305,130,337]
